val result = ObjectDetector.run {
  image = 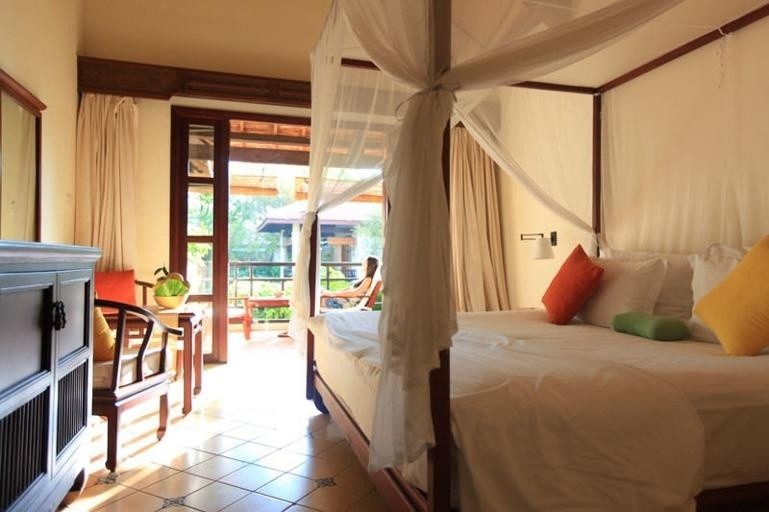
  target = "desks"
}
[103,306,206,415]
[251,294,291,308]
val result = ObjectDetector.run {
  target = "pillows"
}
[95,269,136,312]
[682,254,744,343]
[575,254,667,328]
[612,311,691,340]
[541,242,604,325]
[610,250,694,323]
[708,244,754,262]
[692,232,769,356]
[93,291,116,362]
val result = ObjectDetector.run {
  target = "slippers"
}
[277,331,290,338]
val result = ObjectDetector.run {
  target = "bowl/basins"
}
[154,296,188,309]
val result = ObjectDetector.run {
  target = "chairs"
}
[318,280,382,312]
[92,269,182,381]
[94,297,185,471]
[200,296,251,339]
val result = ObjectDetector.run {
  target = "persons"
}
[278,257,378,337]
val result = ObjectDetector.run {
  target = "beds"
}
[306,1,769,512]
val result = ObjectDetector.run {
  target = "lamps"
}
[532,231,561,260]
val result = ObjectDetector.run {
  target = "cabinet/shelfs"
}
[0,241,105,512]
